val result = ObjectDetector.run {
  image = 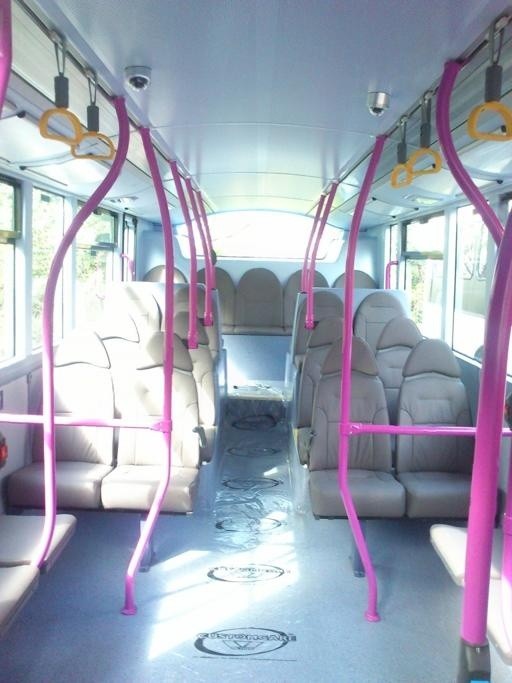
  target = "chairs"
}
[394,339,472,518]
[0,327,115,515]
[87,285,221,464]
[308,335,405,576]
[290,290,423,467]
[100,332,205,571]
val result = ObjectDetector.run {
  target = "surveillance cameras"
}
[367,92,390,116]
[126,67,152,91]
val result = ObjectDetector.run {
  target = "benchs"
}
[142,265,379,336]
[430,524,510,662]
[2,513,76,635]
